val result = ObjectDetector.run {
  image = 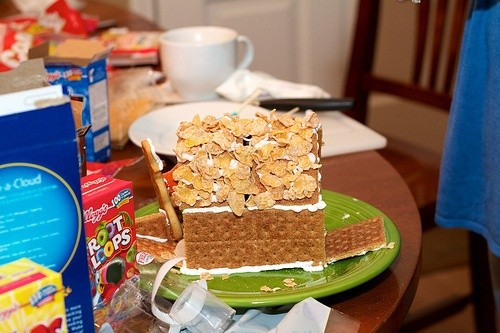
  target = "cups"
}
[159,26,254,101]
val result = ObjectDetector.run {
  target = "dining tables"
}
[0,0,424,333]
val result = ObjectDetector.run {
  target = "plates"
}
[130,189,401,308]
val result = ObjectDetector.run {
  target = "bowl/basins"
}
[129,100,278,163]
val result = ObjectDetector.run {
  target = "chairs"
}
[341,0,500,333]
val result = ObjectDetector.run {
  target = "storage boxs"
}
[0,38,142,333]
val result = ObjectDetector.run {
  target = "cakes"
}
[135,108,328,280]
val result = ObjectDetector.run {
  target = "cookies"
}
[326,215,395,264]
